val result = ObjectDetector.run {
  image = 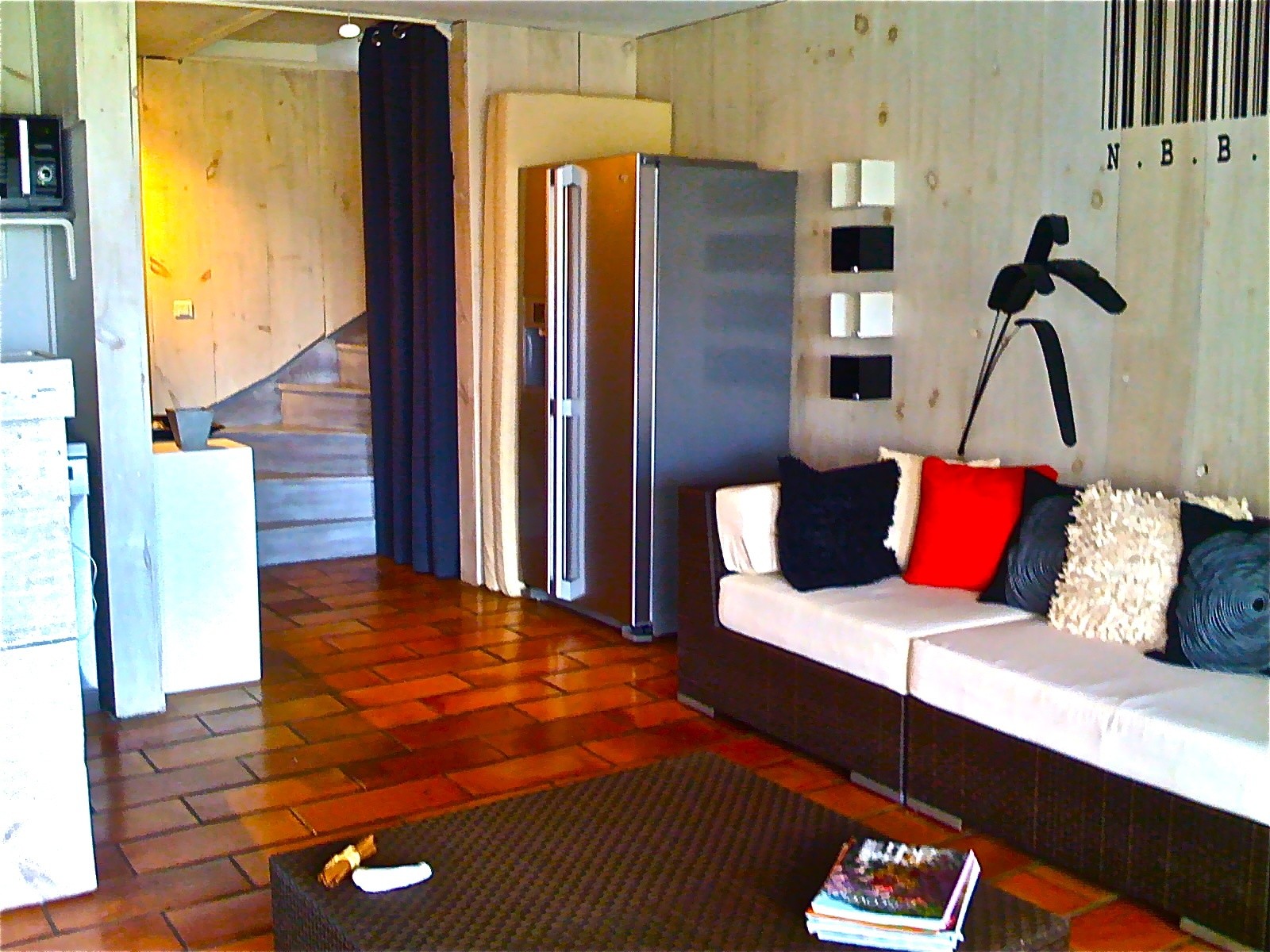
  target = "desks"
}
[152,437,261,694]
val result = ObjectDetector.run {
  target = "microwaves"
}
[0,112,66,212]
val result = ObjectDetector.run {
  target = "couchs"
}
[676,471,1270,952]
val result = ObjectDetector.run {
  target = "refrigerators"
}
[516,152,800,645]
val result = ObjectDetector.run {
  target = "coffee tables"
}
[266,752,1071,952]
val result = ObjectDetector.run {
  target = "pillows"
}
[1048,479,1252,651]
[776,453,900,591]
[903,455,1058,593]
[973,469,1083,617]
[875,446,1003,570]
[1143,499,1270,676]
